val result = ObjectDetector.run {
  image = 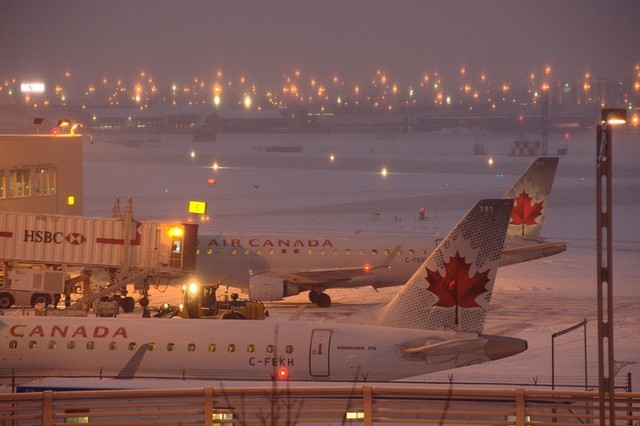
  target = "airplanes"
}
[78,157,567,313]
[0,199,528,381]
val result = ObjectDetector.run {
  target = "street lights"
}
[597,109,626,426]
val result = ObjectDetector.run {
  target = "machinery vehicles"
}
[154,284,269,319]
